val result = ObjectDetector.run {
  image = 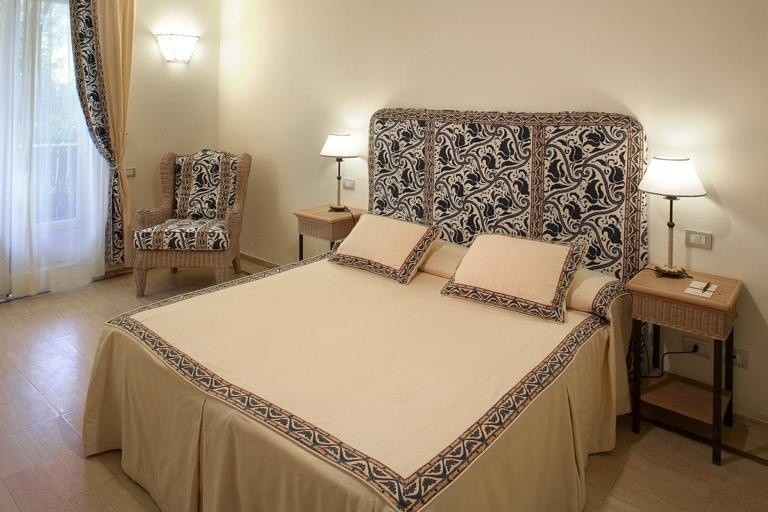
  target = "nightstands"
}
[293,204,365,261]
[626,261,743,466]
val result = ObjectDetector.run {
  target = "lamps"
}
[636,156,708,279]
[150,31,201,65]
[319,133,360,213]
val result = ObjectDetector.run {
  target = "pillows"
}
[327,210,591,325]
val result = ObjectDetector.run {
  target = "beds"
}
[82,107,649,512]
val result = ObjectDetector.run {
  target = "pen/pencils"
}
[702,282,711,294]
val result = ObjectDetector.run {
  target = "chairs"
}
[130,145,252,299]
